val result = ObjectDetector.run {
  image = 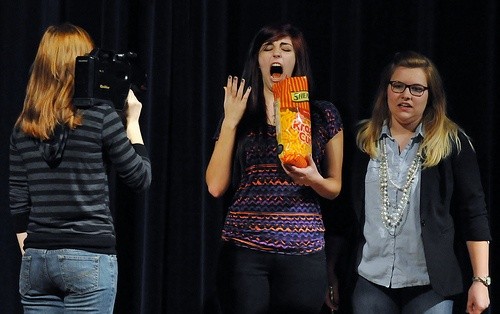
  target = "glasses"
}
[388,80,428,97]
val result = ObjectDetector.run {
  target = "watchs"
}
[472,276,491,287]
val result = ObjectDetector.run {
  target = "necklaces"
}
[380,143,420,237]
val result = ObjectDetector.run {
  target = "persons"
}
[9,22,151,314]
[325,50,491,313]
[205,23,343,314]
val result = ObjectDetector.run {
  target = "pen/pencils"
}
[328,281,337,314]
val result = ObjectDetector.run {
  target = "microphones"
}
[116,52,138,58]
